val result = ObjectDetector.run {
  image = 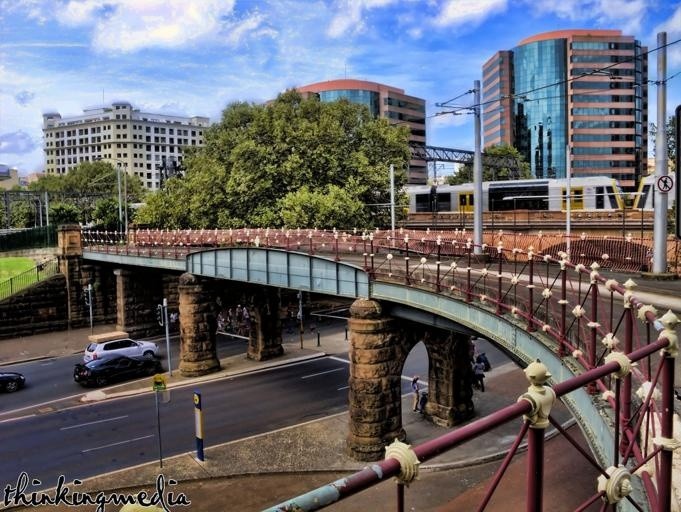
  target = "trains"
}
[398,173,677,213]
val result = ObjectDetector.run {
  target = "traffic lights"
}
[83,289,89,305]
[155,304,163,326]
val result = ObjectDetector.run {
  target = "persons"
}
[473,356,487,392]
[170,302,320,341]
[411,375,420,412]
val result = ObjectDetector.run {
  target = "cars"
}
[0,369,25,395]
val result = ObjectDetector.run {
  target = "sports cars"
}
[68,353,163,387]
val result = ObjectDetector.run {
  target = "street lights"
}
[92,157,128,235]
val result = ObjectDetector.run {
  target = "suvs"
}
[81,336,161,364]
